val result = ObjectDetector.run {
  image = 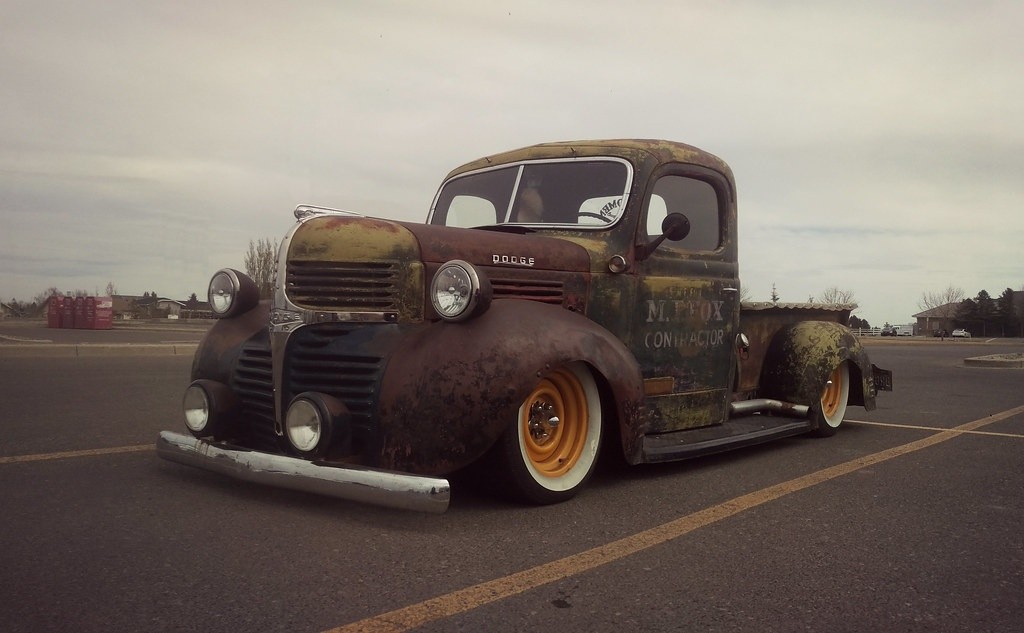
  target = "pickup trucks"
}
[156,139,893,512]
